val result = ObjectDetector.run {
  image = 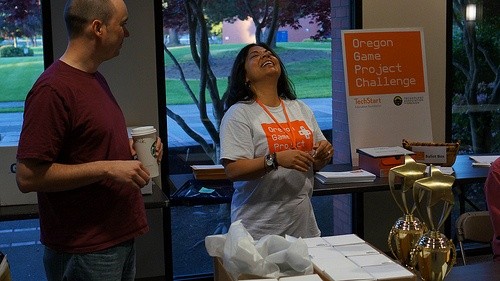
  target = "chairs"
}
[455,212,491,265]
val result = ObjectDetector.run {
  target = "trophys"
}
[387,153,455,281]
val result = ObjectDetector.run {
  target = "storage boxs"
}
[359,151,425,177]
[212,236,417,281]
[0,142,38,205]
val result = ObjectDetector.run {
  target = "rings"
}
[327,151,331,154]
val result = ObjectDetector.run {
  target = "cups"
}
[130,126,159,178]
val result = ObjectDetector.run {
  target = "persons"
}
[485,157,500,281]
[218,43,334,242]
[17,0,164,281]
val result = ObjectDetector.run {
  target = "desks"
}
[445,261,500,281]
[0,181,170,222]
[164,155,491,281]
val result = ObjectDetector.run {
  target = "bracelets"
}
[264,152,278,174]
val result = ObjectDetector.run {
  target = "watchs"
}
[267,155,273,171]
